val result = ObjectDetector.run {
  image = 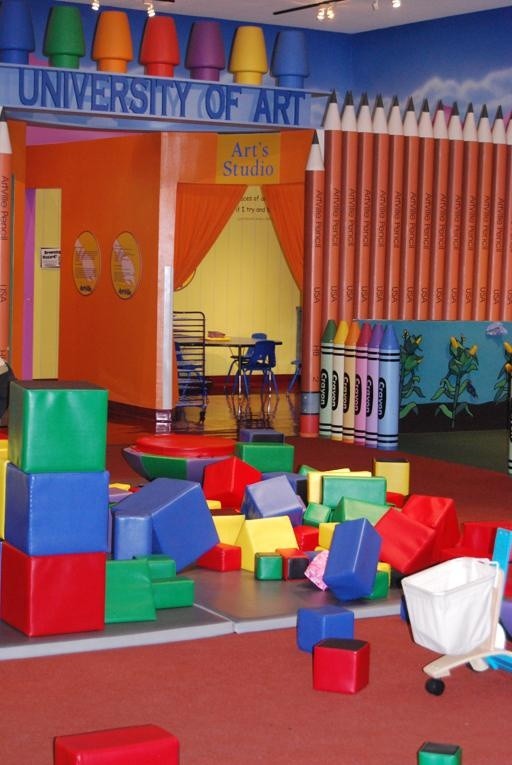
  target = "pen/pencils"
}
[323,88,512,323]
[300,126,325,440]
[318,319,400,451]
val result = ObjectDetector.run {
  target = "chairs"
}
[176,345,208,402]
[225,333,303,399]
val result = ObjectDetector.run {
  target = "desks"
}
[173,336,282,398]
